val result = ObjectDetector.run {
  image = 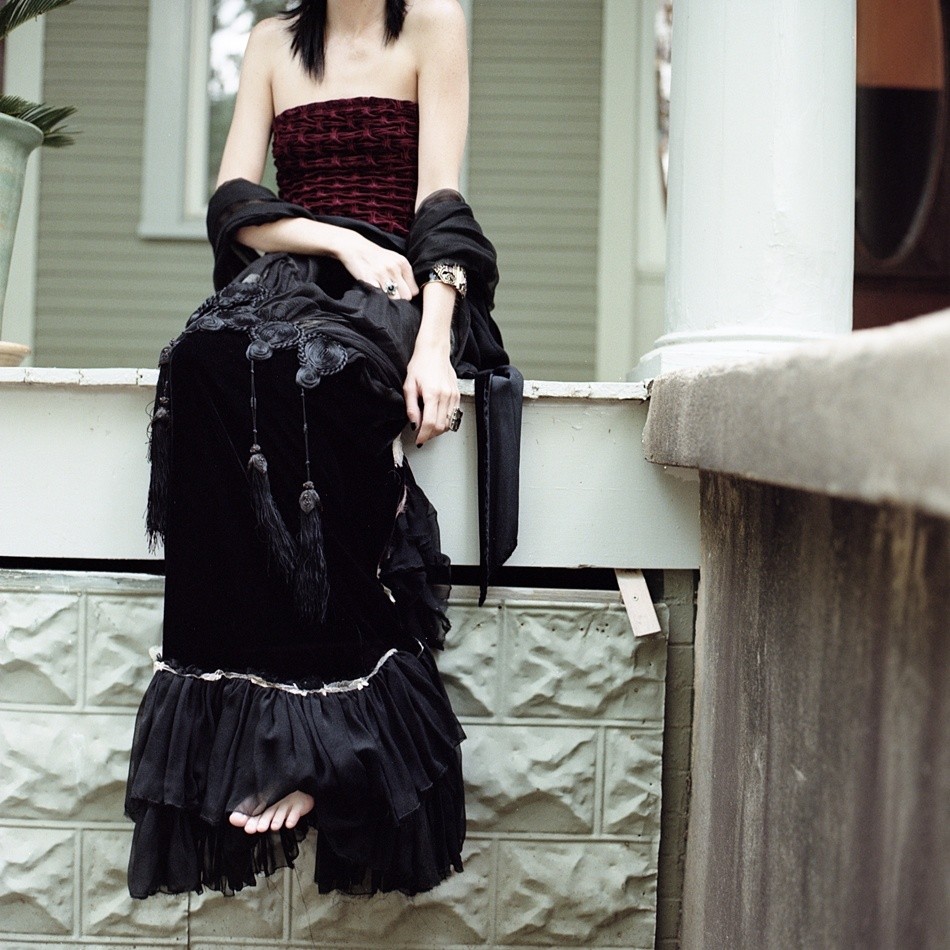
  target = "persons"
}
[123,0,523,900]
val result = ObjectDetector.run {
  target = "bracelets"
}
[419,263,467,303]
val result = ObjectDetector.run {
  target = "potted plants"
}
[0,0,84,340]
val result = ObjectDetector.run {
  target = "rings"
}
[446,408,463,432]
[386,281,398,295]
